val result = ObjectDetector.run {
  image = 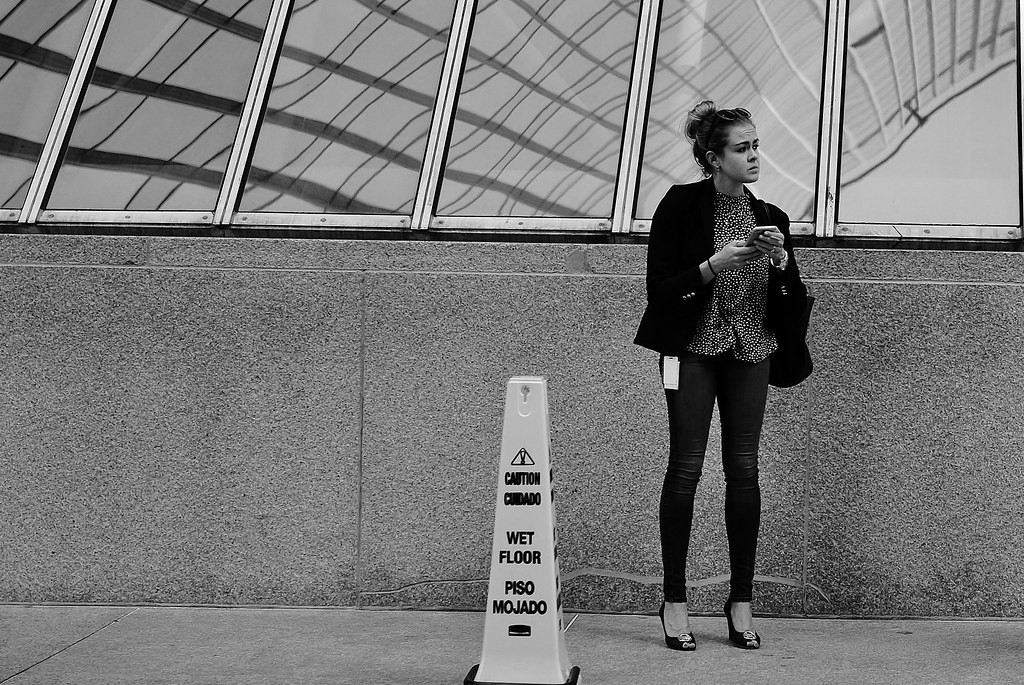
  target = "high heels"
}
[659,600,696,651]
[724,602,760,649]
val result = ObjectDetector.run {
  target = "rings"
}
[771,248,775,254]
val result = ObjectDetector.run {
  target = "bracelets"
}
[707,258,719,276]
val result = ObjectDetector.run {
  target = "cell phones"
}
[743,226,778,247]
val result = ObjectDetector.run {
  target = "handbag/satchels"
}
[758,199,815,388]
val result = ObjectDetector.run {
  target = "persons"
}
[633,101,815,651]
